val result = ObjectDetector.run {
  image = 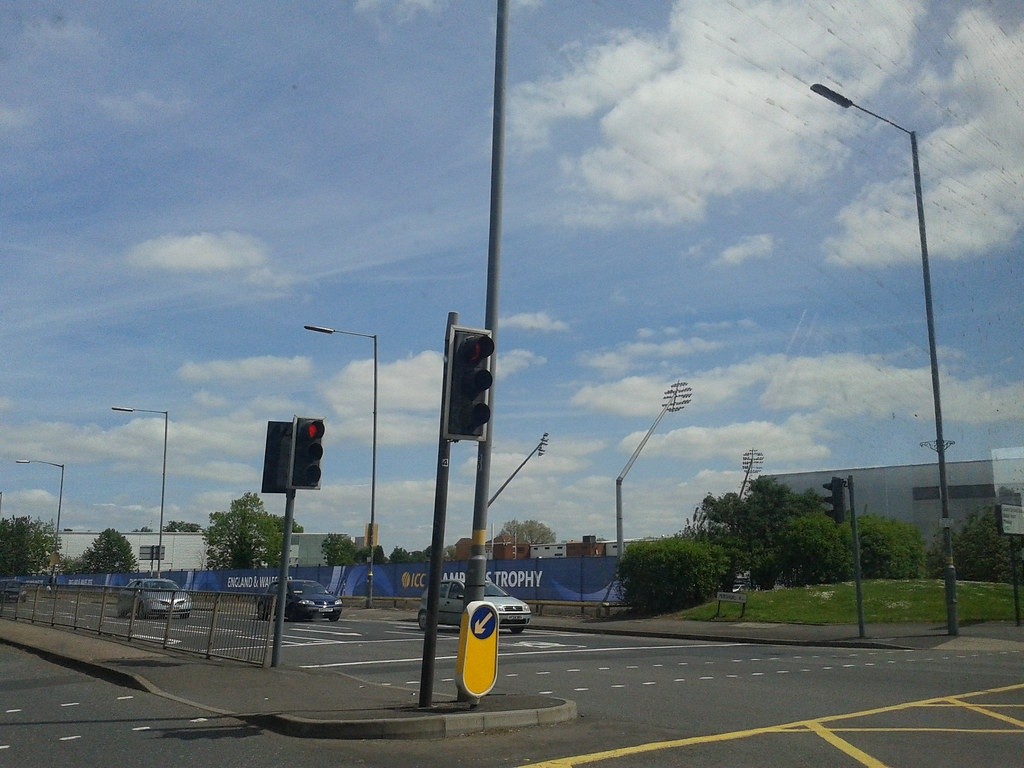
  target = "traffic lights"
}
[440,324,496,442]
[287,413,325,489]
[822,476,846,525]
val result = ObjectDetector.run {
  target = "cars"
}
[118,578,193,620]
[0,580,29,605]
[417,577,532,633]
[257,580,344,621]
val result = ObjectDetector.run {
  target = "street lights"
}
[615,380,694,564]
[487,430,548,507]
[811,82,960,637]
[16,459,65,548]
[112,404,170,579]
[738,446,765,499]
[305,322,379,610]
